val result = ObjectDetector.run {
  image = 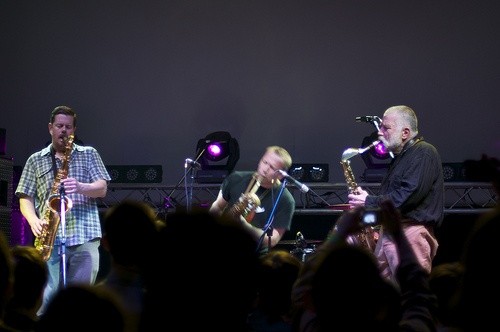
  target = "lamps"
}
[105,164,164,184]
[443,162,464,182]
[285,163,330,183]
[195,131,240,184]
[361,130,396,183]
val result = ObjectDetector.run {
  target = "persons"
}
[210,147,295,247]
[0,200,500,332]
[350,106,444,286]
[14,106,112,316]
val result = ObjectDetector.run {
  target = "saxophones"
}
[228,175,263,223]
[339,141,380,251]
[33,134,75,261]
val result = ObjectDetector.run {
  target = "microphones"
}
[298,231,307,247]
[187,159,201,169]
[356,116,378,123]
[278,170,309,193]
